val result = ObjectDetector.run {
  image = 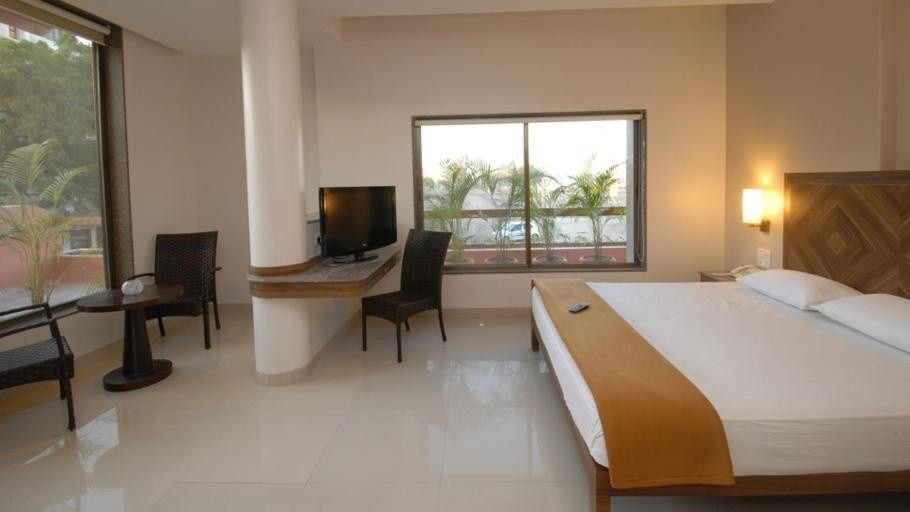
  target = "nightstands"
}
[698,270,736,282]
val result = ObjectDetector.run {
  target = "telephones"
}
[730,265,764,278]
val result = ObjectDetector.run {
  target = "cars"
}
[490,219,542,241]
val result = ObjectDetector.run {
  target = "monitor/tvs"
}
[318,185,397,264]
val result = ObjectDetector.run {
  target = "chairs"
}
[0,303,75,431]
[126,231,222,349]
[362,228,453,362]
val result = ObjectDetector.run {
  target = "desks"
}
[76,284,184,392]
[248,244,402,390]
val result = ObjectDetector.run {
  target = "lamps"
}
[741,188,770,233]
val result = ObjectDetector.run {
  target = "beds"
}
[529,170,910,512]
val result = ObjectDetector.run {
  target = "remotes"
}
[569,303,590,312]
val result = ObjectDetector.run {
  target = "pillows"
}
[810,293,909,354]
[736,268,861,313]
